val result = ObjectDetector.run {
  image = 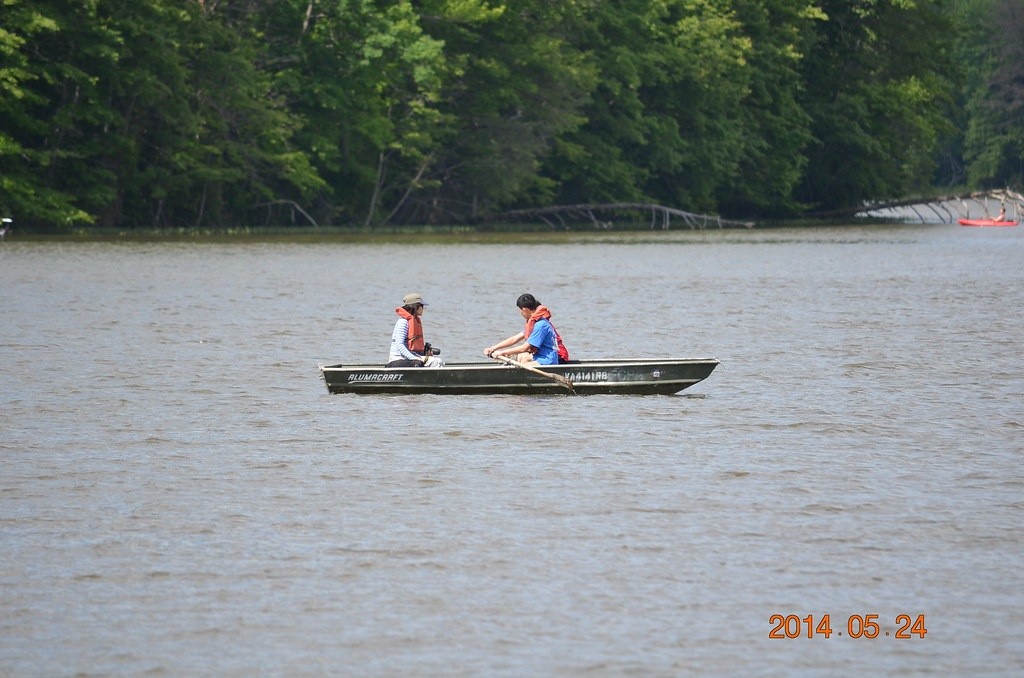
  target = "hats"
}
[403,293,429,308]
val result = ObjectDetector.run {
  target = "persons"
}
[484,293,558,365]
[990,207,1006,222]
[536,300,568,363]
[387,293,444,367]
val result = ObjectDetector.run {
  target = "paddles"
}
[489,352,574,391]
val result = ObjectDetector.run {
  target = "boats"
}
[958,217,1020,227]
[318,357,725,397]
